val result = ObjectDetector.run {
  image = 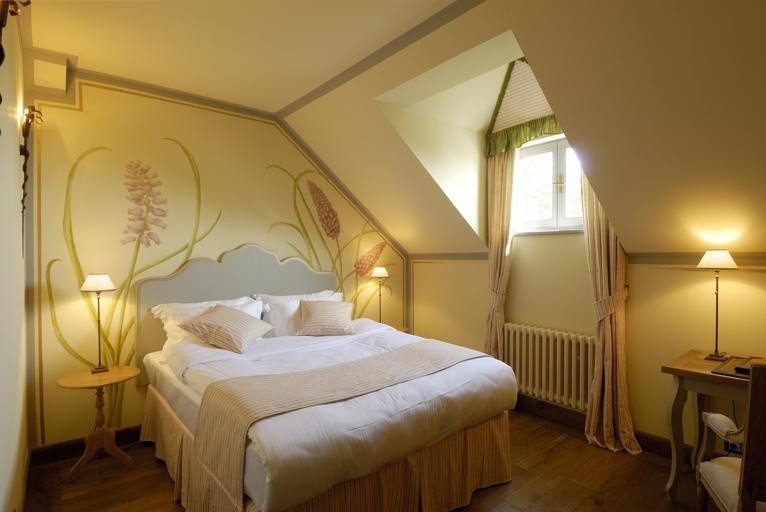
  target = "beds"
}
[135,243,519,512]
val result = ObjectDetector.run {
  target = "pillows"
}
[149,289,357,354]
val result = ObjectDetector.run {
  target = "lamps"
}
[79,274,118,374]
[696,249,739,362]
[370,265,389,324]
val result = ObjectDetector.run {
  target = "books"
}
[735,356,766,376]
[711,354,753,380]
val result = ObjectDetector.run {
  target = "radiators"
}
[501,320,598,418]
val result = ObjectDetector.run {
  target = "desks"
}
[56,365,143,484]
[660,348,766,512]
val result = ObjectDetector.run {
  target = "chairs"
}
[695,363,766,512]
